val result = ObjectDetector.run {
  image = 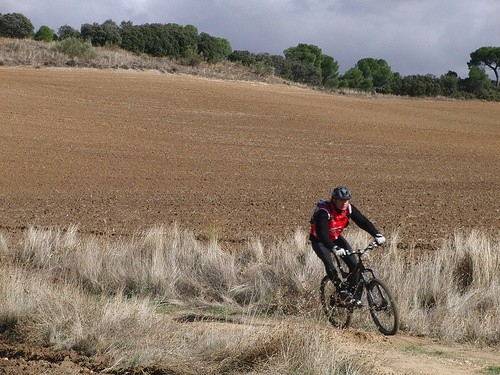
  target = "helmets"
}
[333,187,351,200]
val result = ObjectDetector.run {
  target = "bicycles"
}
[320,240,399,336]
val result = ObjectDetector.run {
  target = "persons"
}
[309,186,387,304]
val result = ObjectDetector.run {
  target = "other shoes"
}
[340,291,352,303]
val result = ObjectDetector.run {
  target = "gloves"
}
[375,234,385,246]
[332,246,346,259]
